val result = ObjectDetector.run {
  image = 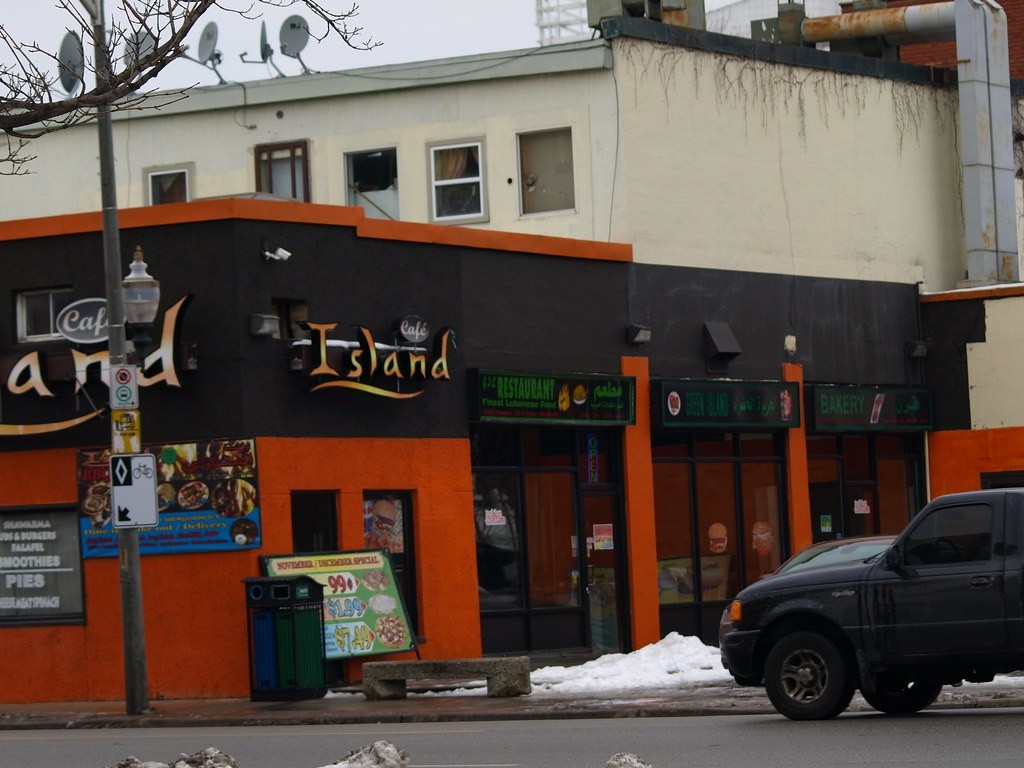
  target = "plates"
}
[667,391,681,415]
[211,479,256,516]
[81,494,107,515]
[87,482,111,495]
[156,483,176,511]
[361,570,390,591]
[177,481,209,510]
[368,594,397,614]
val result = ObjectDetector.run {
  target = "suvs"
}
[720,487,1024,720]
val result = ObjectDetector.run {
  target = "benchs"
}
[363,655,533,698]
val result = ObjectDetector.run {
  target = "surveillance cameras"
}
[275,247,291,261]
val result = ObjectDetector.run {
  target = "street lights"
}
[81,0,160,715]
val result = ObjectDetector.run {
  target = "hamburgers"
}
[372,499,396,532]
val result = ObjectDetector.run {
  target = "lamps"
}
[907,340,929,357]
[703,321,742,375]
[624,323,653,344]
[249,313,281,336]
[120,244,161,365]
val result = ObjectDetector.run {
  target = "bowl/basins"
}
[574,399,586,404]
[230,518,258,544]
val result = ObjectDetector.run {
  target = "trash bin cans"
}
[243,574,328,704]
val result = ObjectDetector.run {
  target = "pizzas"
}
[375,614,406,646]
[362,570,391,591]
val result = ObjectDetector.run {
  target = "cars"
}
[718,536,899,686]
